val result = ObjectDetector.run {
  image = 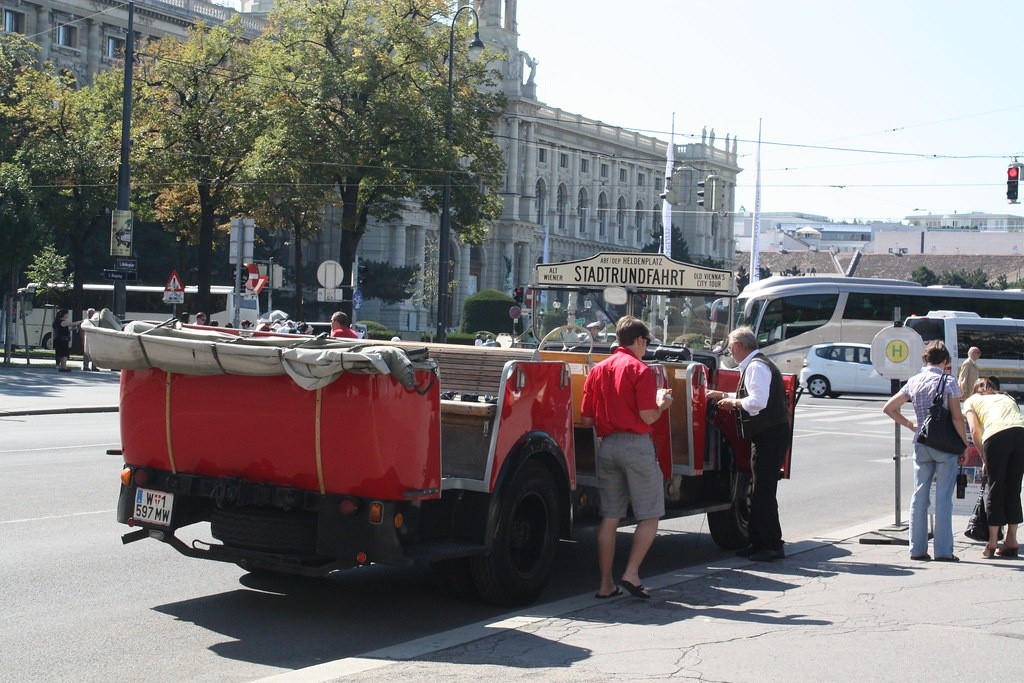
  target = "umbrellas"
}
[260,309,290,324]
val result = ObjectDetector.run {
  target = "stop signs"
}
[245,262,260,290]
[525,287,539,308]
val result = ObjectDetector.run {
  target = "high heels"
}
[983,544,996,558]
[996,543,1018,557]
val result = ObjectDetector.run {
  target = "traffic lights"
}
[513,286,523,303]
[1006,164,1019,200]
[696,175,712,209]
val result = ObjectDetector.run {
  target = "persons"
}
[554,298,561,311]
[225,323,234,328]
[540,308,545,314]
[475,334,483,346]
[957,346,981,402]
[962,376,1024,559]
[255,318,314,336]
[330,310,365,338]
[180,311,191,325]
[705,324,791,561]
[437,322,445,342]
[580,316,674,599]
[584,297,592,313]
[80,308,101,372]
[485,333,496,347]
[391,336,400,342]
[241,319,251,335]
[52,308,83,372]
[583,321,605,343]
[883,339,969,562]
[208,320,218,327]
[191,312,207,325]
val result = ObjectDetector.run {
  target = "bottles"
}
[956,474,967,499]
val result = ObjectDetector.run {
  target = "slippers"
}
[595,587,623,598]
[621,580,649,598]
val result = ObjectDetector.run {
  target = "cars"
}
[79,250,812,611]
[798,341,903,400]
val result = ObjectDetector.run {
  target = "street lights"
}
[434,5,485,341]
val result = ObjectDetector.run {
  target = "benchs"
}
[541,348,671,430]
[303,341,539,417]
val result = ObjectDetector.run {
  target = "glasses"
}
[637,336,651,347]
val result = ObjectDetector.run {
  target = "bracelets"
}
[723,391,725,398]
[732,399,737,410]
[906,421,909,427]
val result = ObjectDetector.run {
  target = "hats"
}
[587,321,602,330]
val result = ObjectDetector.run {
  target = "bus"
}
[903,311,1024,403]
[708,274,1024,379]
[12,280,261,350]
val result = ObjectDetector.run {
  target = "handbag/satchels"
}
[917,374,967,454]
[965,474,1004,542]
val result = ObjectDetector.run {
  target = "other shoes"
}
[748,547,784,561]
[935,555,959,562]
[93,369,99,371]
[736,546,756,557]
[82,367,90,371]
[910,553,931,560]
[59,368,71,372]
